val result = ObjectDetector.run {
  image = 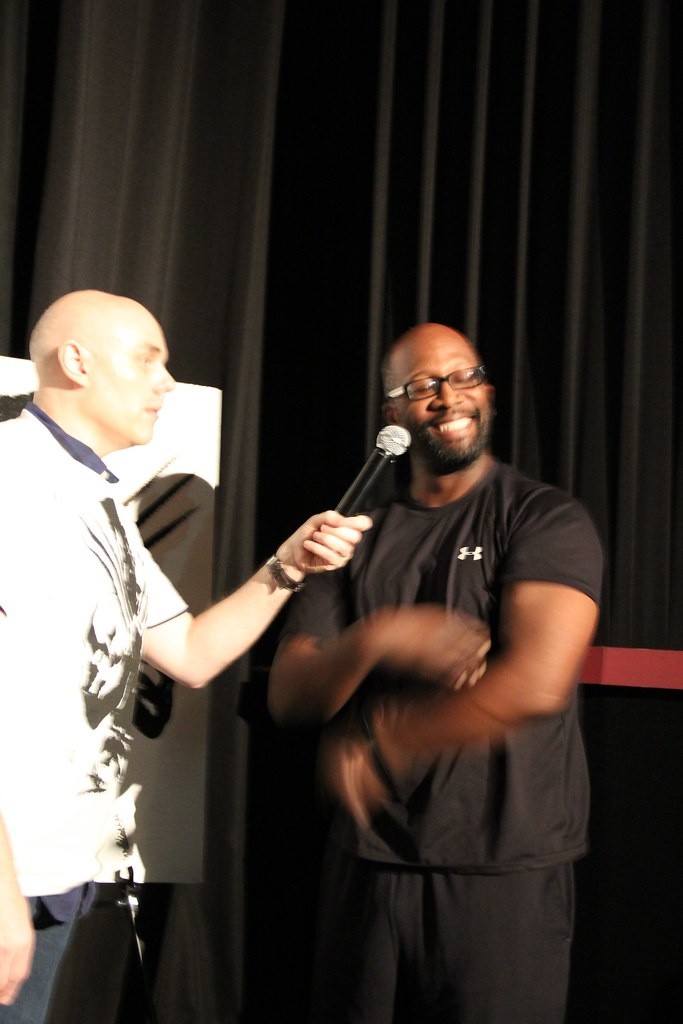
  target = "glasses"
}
[385,365,487,401]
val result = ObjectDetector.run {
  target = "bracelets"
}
[359,708,406,807]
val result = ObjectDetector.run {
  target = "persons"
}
[267,322,603,1024]
[0,289,373,1024]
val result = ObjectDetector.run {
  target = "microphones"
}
[331,424,411,518]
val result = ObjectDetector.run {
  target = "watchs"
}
[266,553,308,592]
